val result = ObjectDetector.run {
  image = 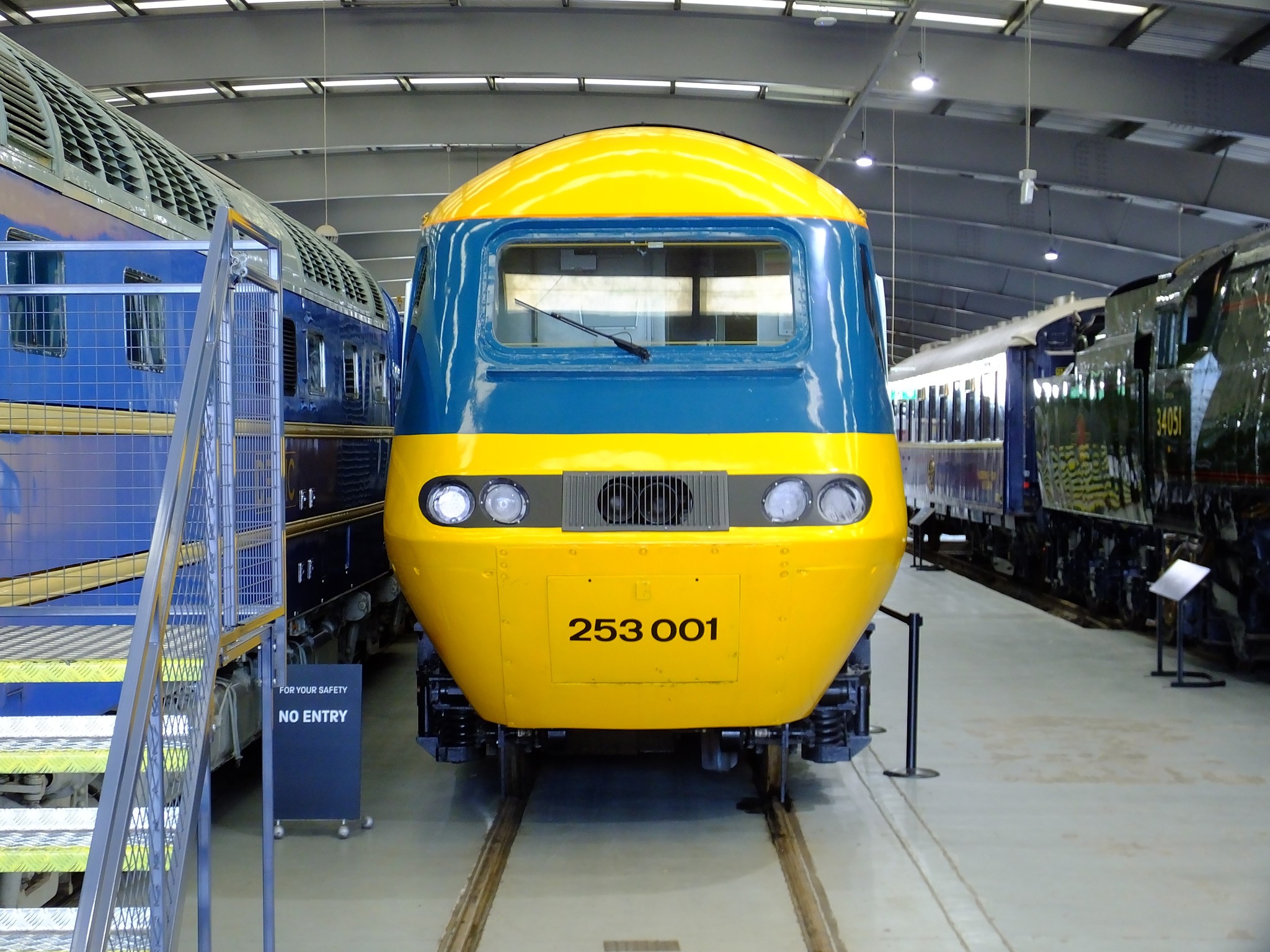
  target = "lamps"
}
[314,1,338,244]
[852,106,877,167]
[912,22,938,92]
[1041,185,1060,260]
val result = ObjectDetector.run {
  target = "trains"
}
[379,125,908,808]
[0,31,404,914]
[885,228,1270,685]
